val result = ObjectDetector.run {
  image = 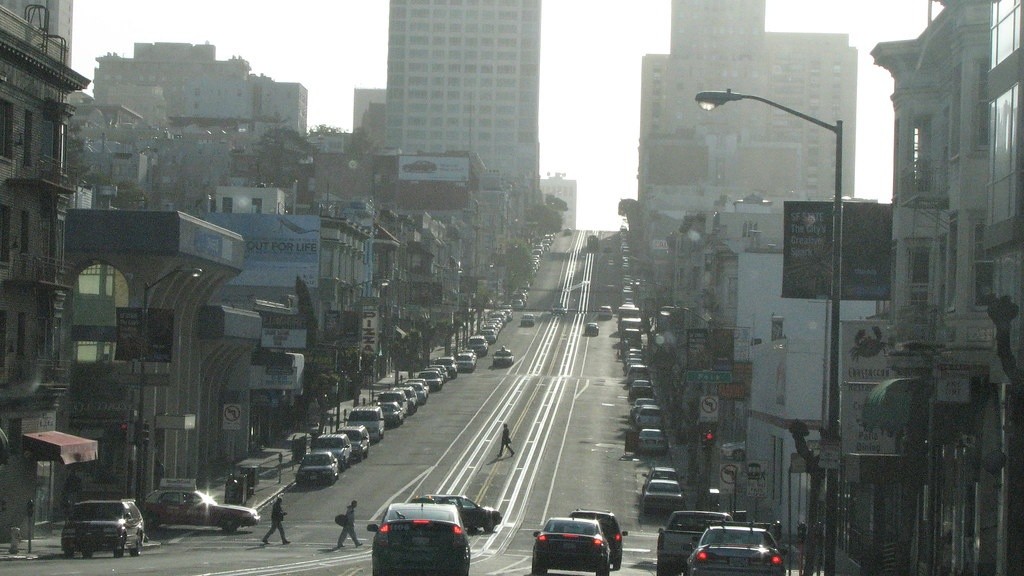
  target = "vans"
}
[342,404,385,445]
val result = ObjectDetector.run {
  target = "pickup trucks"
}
[655,510,734,576]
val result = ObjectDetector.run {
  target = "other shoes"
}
[263,539,270,545]
[283,541,290,544]
[338,544,345,548]
[511,452,515,457]
[497,453,502,457]
[356,543,364,547]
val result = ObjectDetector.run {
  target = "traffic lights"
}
[134,421,151,447]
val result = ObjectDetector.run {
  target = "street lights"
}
[658,303,714,512]
[408,266,463,380]
[633,278,685,447]
[693,88,845,576]
[132,266,204,507]
[334,277,391,431]
[464,261,496,348]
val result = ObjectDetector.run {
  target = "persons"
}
[338,500,363,548]
[262,497,291,545]
[497,423,515,457]
[154,456,169,490]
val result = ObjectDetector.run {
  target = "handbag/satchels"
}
[335,513,348,526]
[503,438,511,444]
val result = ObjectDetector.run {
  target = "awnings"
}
[0,428,10,465]
[863,377,918,426]
[23,430,98,465]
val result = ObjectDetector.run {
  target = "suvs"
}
[336,425,370,464]
[59,499,146,558]
[312,433,352,472]
[570,508,629,570]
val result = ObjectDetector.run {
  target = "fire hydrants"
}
[7,526,22,555]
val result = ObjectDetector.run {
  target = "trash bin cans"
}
[291,438,306,462]
[241,466,259,487]
[226,474,247,506]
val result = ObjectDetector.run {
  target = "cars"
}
[640,466,681,495]
[294,450,339,488]
[634,428,668,455]
[531,516,614,576]
[685,519,786,576]
[411,493,503,533]
[138,478,261,533]
[374,224,663,429]
[366,501,472,576]
[642,479,685,513]
[720,440,746,462]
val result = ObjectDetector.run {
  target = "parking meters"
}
[329,416,334,433]
[277,452,284,484]
[26,499,36,554]
[343,409,347,426]
